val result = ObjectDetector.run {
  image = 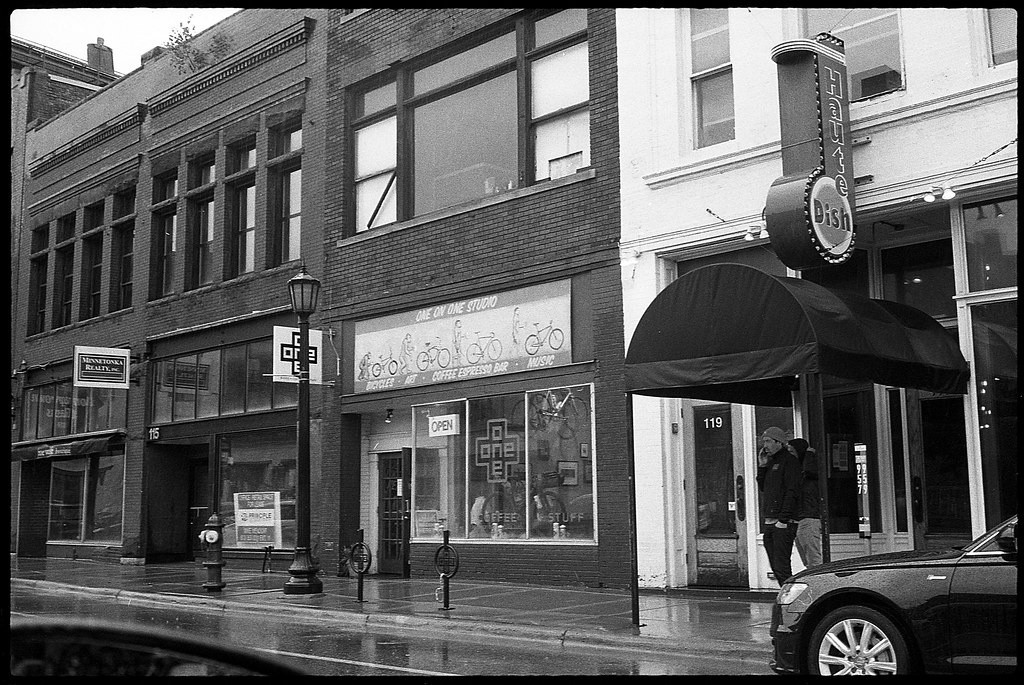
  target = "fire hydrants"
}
[198,513,228,591]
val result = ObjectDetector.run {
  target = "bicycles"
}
[481,472,567,535]
[529,388,588,434]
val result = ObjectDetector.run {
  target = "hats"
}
[762,427,784,443]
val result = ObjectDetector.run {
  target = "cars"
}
[769,514,1017,676]
[526,493,593,539]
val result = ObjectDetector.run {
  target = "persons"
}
[470,486,492,530]
[755,426,801,591]
[785,438,823,568]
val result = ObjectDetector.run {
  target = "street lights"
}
[287,255,322,594]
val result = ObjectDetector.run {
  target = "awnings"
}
[621,262,972,407]
